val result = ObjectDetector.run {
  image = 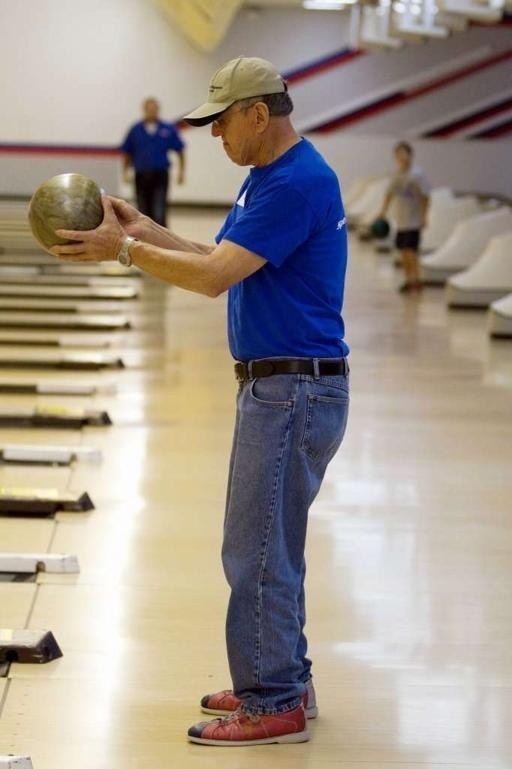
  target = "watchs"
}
[118,237,134,268]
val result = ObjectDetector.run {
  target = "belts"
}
[235,360,344,378]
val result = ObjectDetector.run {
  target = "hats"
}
[183,58,287,127]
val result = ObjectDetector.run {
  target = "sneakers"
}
[188,678,318,746]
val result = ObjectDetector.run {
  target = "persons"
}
[47,55,354,747]
[377,142,430,294]
[121,99,186,227]
